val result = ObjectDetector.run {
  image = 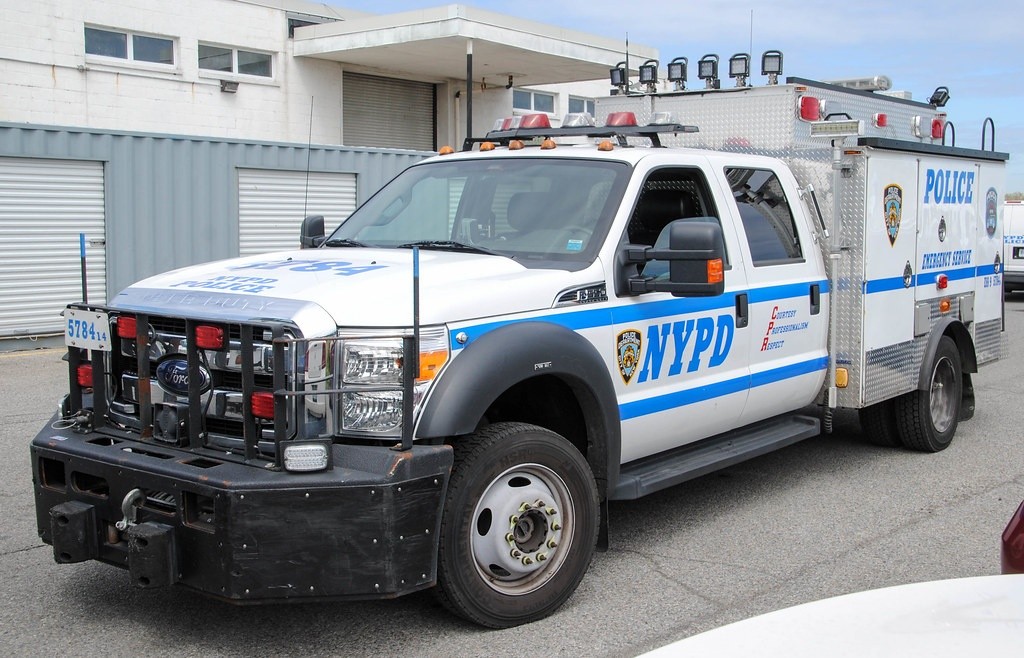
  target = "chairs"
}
[494,191,567,241]
[629,190,696,275]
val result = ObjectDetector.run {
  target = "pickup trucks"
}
[28,49,1011,631]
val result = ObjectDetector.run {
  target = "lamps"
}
[220,79,239,93]
